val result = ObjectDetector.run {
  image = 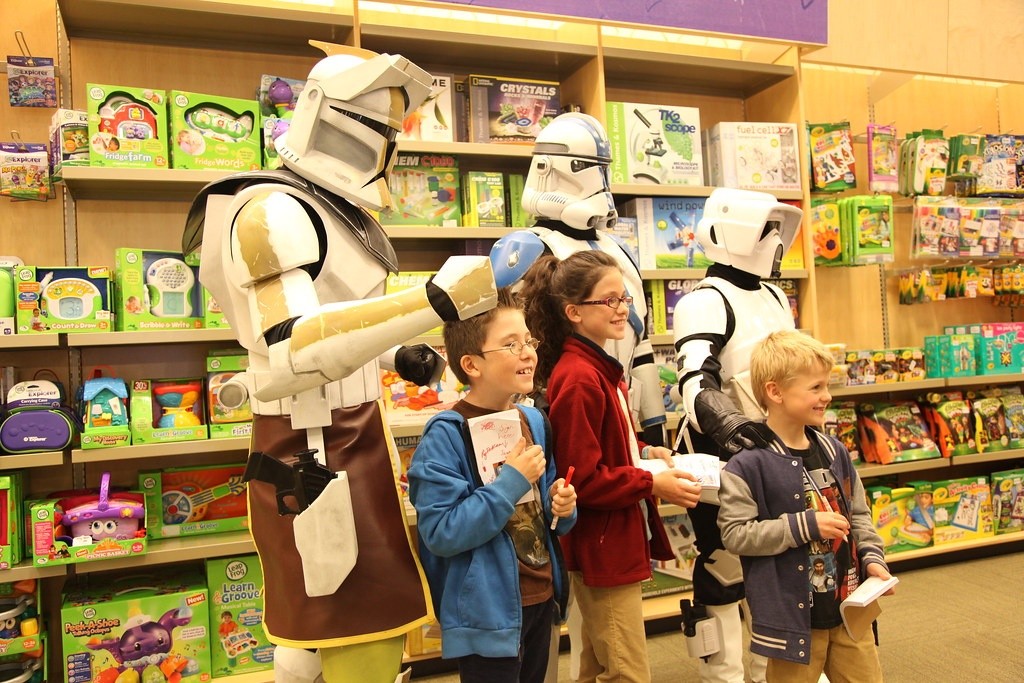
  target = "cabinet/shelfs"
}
[0,0,821,683]
[828,373,1024,564]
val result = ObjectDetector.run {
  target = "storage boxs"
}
[47,76,562,226]
[822,322,1024,557]
[606,98,806,417]
[0,246,468,683]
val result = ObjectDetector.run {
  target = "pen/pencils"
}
[821,496,848,543]
[550,466,575,530]
[671,412,691,456]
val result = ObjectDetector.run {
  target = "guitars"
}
[162,474,247,526]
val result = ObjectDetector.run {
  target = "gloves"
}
[394,346,437,386]
[694,387,773,453]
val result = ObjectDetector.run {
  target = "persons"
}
[218,611,239,639]
[674,188,803,683]
[905,493,934,531]
[487,112,667,683]
[717,332,893,683]
[521,251,702,683]
[182,51,498,683]
[406,288,577,683]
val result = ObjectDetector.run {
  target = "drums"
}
[154,382,202,428]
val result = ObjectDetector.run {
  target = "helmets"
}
[274,52,433,215]
[697,188,802,279]
[521,112,618,230]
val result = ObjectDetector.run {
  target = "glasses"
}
[581,296,634,308]
[473,338,540,355]
